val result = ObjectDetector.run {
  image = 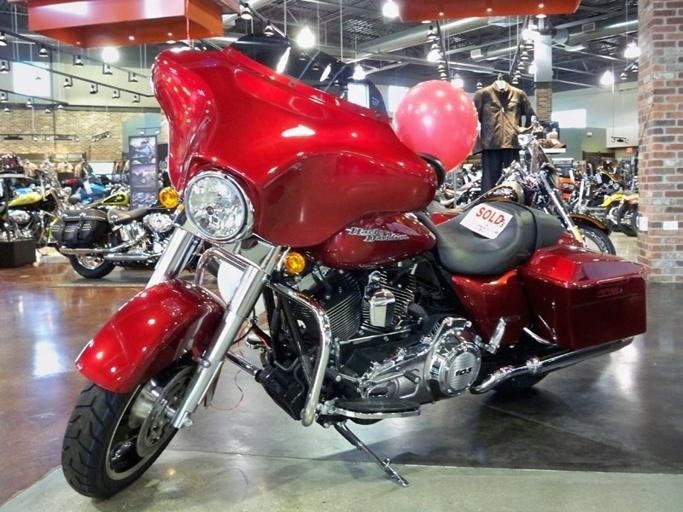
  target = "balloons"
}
[395,78,477,174]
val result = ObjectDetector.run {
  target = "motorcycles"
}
[435,119,639,257]
[1,152,187,280]
[61,2,647,499]
